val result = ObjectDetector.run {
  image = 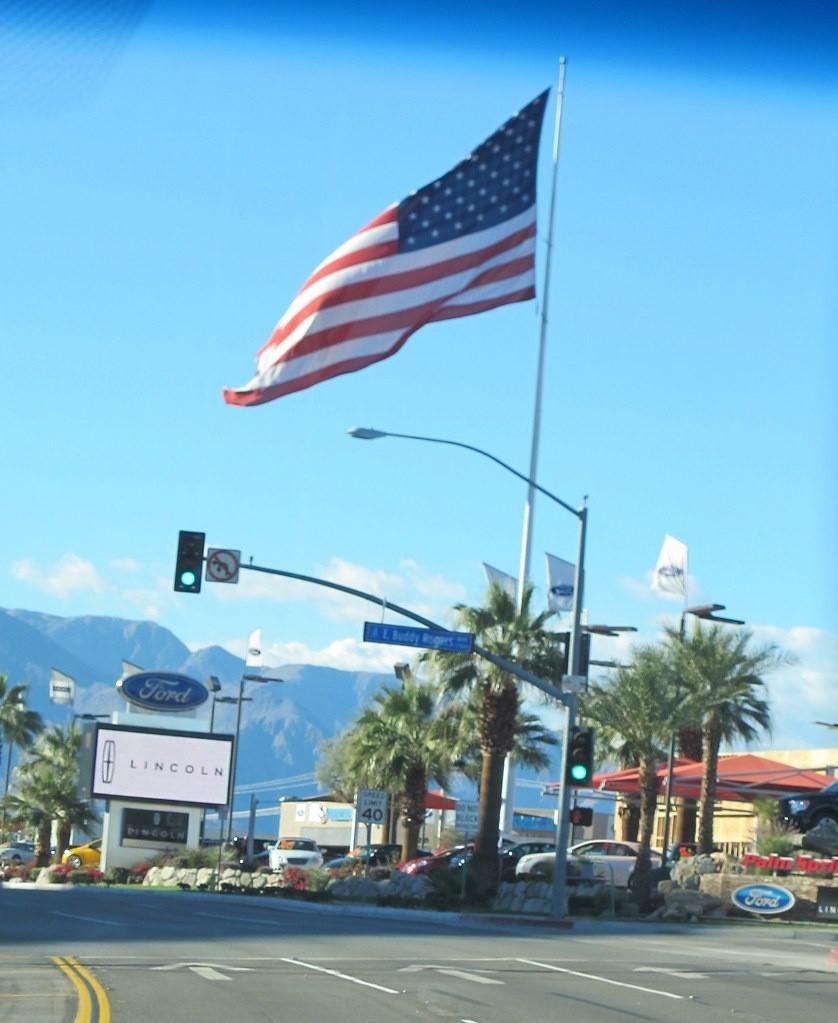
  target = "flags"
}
[546,553,576,611]
[483,563,516,622]
[222,86,532,406]
[245,628,262,667]
[652,536,688,595]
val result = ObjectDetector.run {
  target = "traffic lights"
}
[568,726,594,788]
[174,531,205,592]
[570,807,595,827]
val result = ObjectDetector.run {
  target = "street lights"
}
[346,426,594,920]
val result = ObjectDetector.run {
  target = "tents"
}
[544,754,838,803]
[302,787,454,812]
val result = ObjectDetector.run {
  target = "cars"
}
[0,838,102,871]
[516,839,666,891]
[326,844,434,877]
[199,836,350,878]
[778,781,838,831]
[403,844,476,881]
[448,843,556,879]
